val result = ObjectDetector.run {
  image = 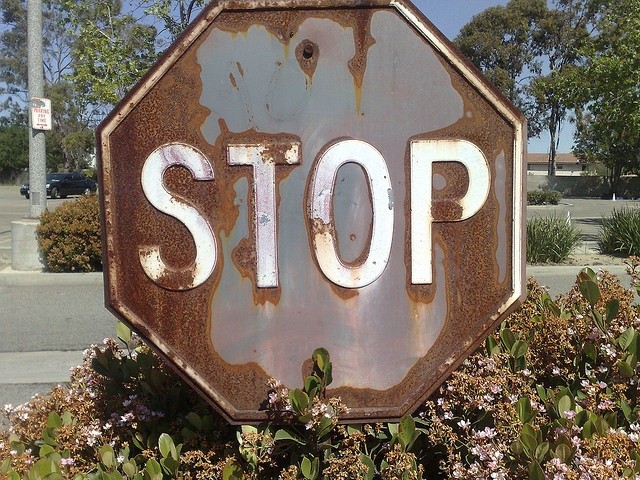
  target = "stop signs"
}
[93,4,527,425]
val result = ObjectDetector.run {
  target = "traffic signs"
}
[31,98,52,131]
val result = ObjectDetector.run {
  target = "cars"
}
[20,173,98,199]
